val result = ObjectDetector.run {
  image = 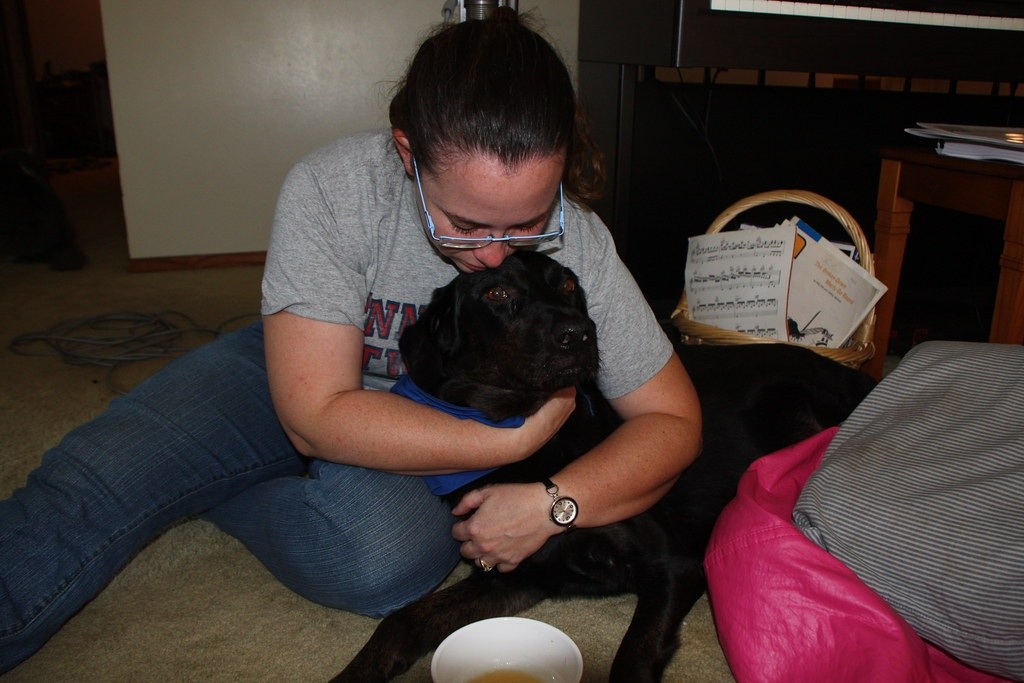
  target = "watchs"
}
[544,479,576,530]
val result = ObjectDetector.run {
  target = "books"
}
[686,209,887,352]
[904,121,1024,166]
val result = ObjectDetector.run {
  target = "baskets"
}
[670,191,876,370]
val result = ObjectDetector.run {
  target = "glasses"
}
[413,156,564,248]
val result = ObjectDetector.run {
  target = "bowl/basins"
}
[430,616,584,683]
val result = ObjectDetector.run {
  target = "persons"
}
[0,15,704,683]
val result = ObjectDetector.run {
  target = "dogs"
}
[329,249,881,683]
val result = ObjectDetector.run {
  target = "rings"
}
[480,557,492,571]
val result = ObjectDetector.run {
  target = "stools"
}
[868,147,1024,377]
